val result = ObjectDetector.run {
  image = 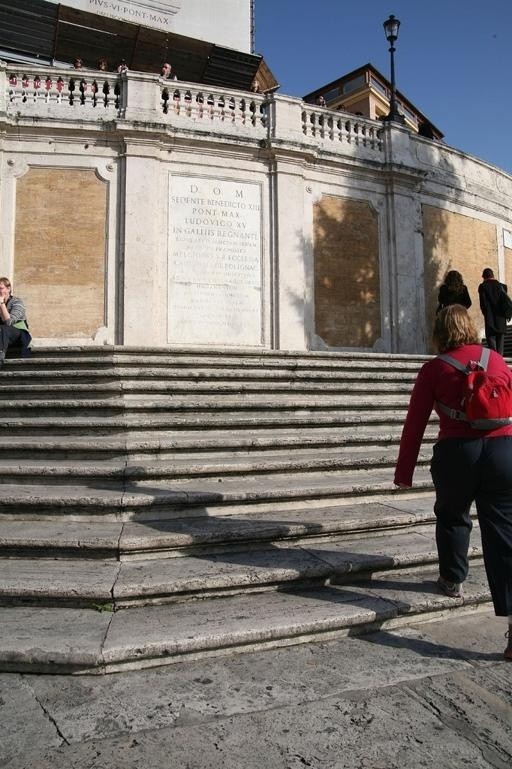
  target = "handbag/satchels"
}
[500,285,512,321]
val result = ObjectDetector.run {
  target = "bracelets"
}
[323,103,326,106]
[0,301,4,305]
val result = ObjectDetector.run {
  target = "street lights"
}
[381,12,405,122]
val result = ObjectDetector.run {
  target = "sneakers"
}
[437,575,464,598]
[0,351,5,365]
[504,630,512,659]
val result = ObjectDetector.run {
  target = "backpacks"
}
[434,346,512,431]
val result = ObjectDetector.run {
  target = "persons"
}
[250,79,263,94]
[435,269,473,315]
[160,62,179,115]
[310,93,327,125]
[111,58,129,110]
[0,277,34,364]
[477,267,512,357]
[91,56,108,108]
[389,303,512,663]
[65,55,86,106]
